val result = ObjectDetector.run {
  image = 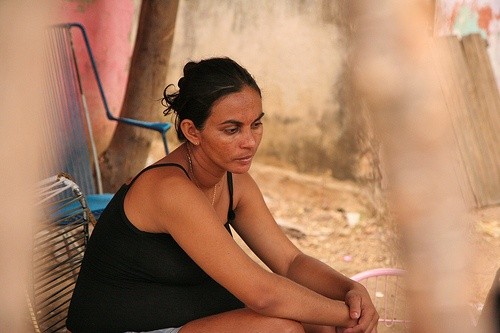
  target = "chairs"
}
[351,267,478,333]
[22,23,172,333]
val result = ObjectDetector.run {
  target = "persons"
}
[62,56,379,333]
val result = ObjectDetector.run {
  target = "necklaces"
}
[186,151,218,207]
